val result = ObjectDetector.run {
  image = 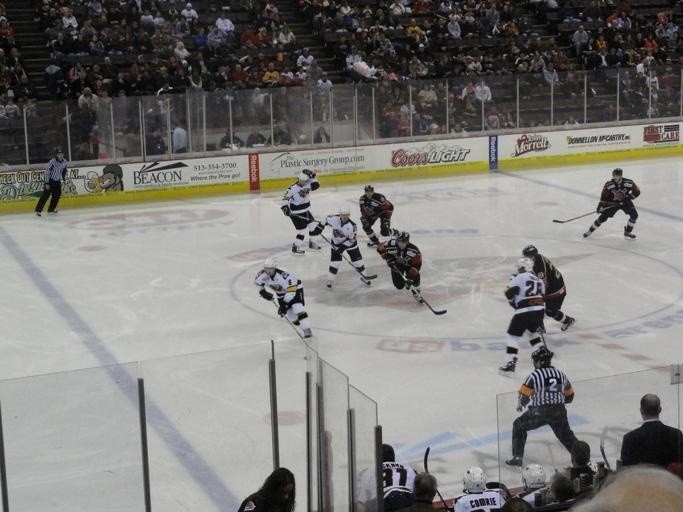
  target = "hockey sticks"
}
[424,447,449,510]
[273,298,312,360]
[553,205,614,224]
[600,428,613,470]
[322,234,377,279]
[392,263,448,315]
[487,481,512,500]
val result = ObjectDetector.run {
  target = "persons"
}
[523,245,574,331]
[280,173,321,254]
[359,185,399,246]
[34,150,69,216]
[377,231,423,304]
[583,169,640,238]
[506,346,592,466]
[499,258,546,372]
[315,206,371,288]
[254,258,312,338]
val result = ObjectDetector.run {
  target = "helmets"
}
[263,258,277,273]
[397,231,410,250]
[462,467,486,494]
[532,346,553,365]
[612,168,623,177]
[340,184,374,216]
[298,172,311,184]
[521,464,546,490]
[517,244,538,273]
[53,146,67,160]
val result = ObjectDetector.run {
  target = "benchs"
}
[1,1,682,166]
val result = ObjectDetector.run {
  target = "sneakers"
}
[367,240,379,247]
[623,226,636,238]
[413,290,424,303]
[505,457,522,467]
[326,277,373,289]
[34,210,58,216]
[302,327,312,339]
[499,356,518,372]
[584,229,593,238]
[291,242,320,253]
[560,316,574,332]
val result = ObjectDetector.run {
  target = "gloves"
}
[259,288,291,317]
[597,198,630,214]
[384,253,396,267]
[299,188,309,197]
[333,243,348,255]
[309,223,325,236]
[281,205,291,217]
